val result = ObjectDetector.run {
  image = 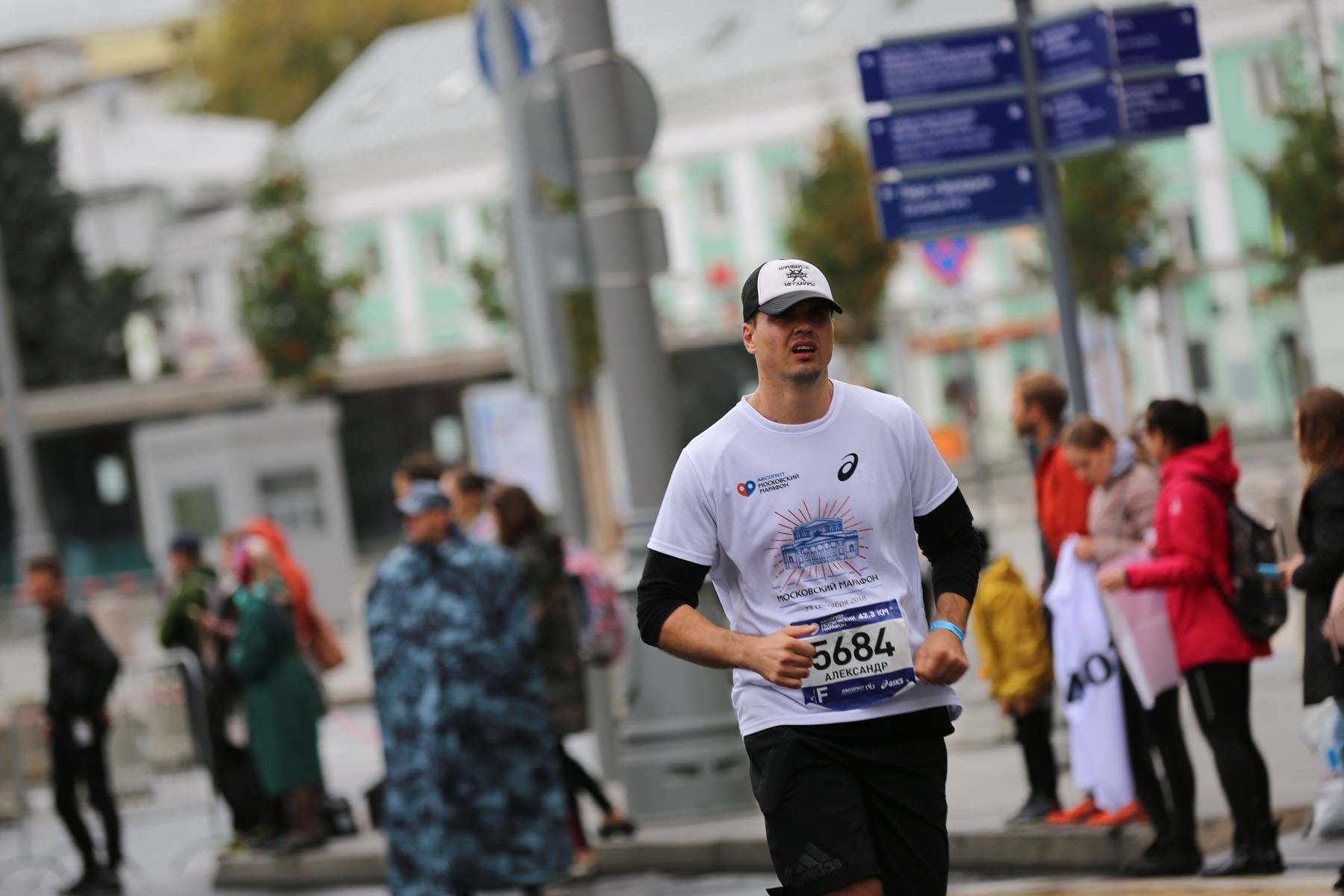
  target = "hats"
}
[168,533,201,564]
[741,259,843,323]
[390,481,449,518]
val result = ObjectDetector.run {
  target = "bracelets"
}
[930,620,965,644]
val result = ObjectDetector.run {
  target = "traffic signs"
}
[1024,5,1110,88]
[873,153,1049,243]
[863,90,1035,172]
[1110,1,1202,73]
[1034,72,1122,167]
[853,18,1028,103]
[1118,62,1213,140]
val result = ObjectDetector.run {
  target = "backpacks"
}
[1183,473,1290,644]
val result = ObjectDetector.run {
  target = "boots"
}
[275,786,331,854]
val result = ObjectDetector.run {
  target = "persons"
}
[23,454,635,895]
[634,259,982,896]
[918,369,1344,896]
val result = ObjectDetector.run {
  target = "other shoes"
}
[1123,838,1204,877]
[1006,797,1060,827]
[1043,800,1103,827]
[599,811,634,838]
[572,850,597,880]
[59,864,123,896]
[1202,820,1286,877]
[1088,801,1149,826]
